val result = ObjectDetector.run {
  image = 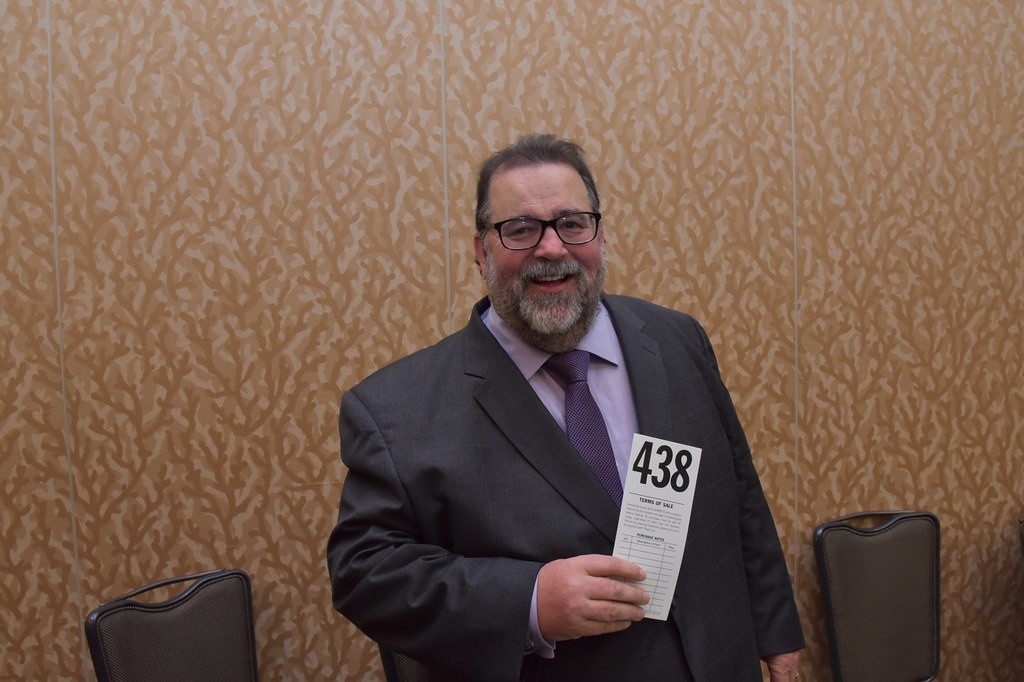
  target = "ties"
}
[543,349,624,511]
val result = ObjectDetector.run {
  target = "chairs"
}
[814,505,941,682]
[84,564,261,682]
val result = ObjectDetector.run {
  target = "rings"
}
[795,672,799,677]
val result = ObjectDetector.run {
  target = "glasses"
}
[484,212,602,251]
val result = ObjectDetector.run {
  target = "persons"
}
[325,133,809,682]
